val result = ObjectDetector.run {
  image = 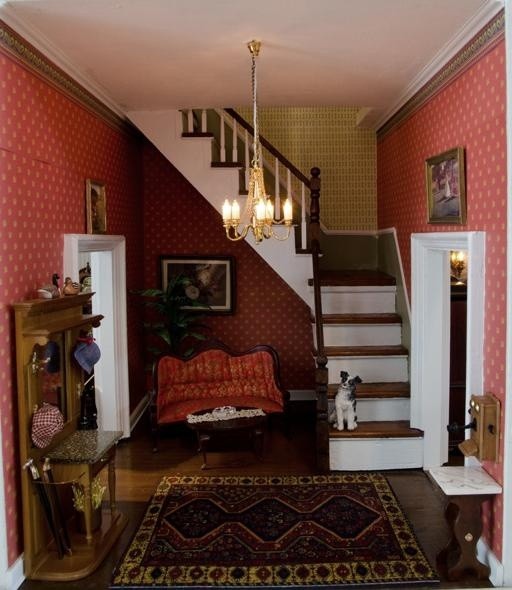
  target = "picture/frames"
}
[158,254,237,317]
[85,178,107,234]
[424,145,467,226]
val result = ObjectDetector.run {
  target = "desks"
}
[8,291,130,581]
[422,465,503,582]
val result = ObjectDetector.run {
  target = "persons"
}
[431,158,458,200]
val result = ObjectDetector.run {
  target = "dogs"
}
[327,371,363,431]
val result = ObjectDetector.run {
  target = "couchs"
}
[152,340,291,453]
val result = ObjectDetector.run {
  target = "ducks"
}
[63,277,82,294]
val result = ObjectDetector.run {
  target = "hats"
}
[74,341,101,375]
[31,403,65,450]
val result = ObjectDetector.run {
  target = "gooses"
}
[37,273,61,299]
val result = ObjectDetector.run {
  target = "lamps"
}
[222,39,294,245]
[451,251,467,278]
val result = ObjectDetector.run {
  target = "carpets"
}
[112,470,440,590]
